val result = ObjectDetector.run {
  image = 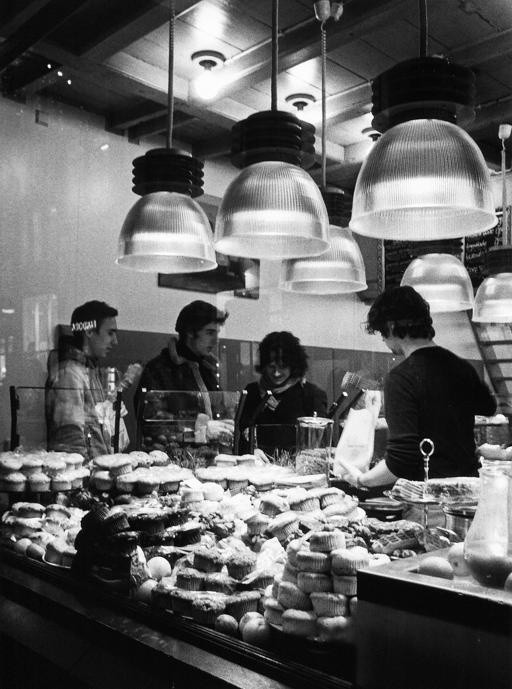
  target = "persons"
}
[234,331,328,456]
[45,300,144,465]
[133,299,236,468]
[328,285,497,492]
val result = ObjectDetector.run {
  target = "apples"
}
[446,542,473,578]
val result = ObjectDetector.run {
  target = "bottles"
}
[461,457,512,586]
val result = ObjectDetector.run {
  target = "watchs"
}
[356,476,371,492]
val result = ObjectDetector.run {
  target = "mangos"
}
[417,554,452,581]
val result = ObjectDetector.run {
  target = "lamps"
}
[349,1,500,242]
[115,1,218,276]
[398,239,475,315]
[213,1,331,263]
[278,1,367,295]
[470,122,512,323]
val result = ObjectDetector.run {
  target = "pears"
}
[462,548,512,590]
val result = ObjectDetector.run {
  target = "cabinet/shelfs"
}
[0,537,512,689]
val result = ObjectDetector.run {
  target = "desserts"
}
[1,443,390,640]
